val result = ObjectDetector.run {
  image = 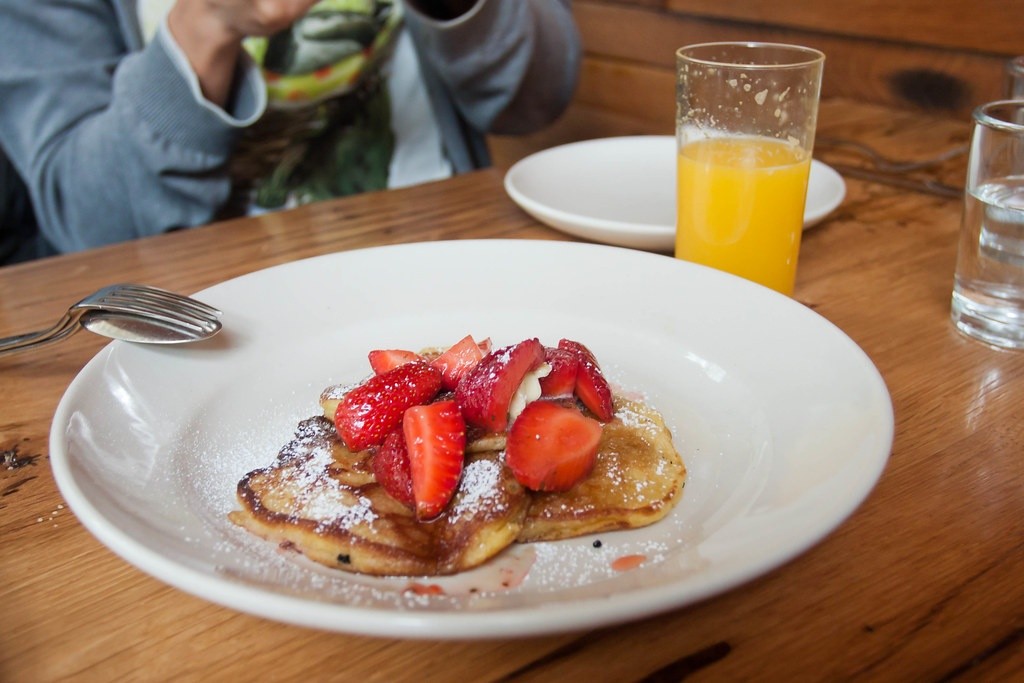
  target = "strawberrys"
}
[330,337,616,520]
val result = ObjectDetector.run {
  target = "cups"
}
[951,100,1024,350]
[1004,55,1024,175]
[675,41,825,300]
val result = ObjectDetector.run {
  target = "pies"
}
[238,383,686,577]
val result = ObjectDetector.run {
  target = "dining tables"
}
[0,103,1024,683]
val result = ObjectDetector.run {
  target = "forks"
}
[1,282,223,351]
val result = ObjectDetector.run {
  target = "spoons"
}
[0,310,223,356]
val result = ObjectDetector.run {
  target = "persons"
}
[0,0,581,263]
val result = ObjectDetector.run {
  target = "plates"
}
[47,238,894,636]
[503,136,847,249]
